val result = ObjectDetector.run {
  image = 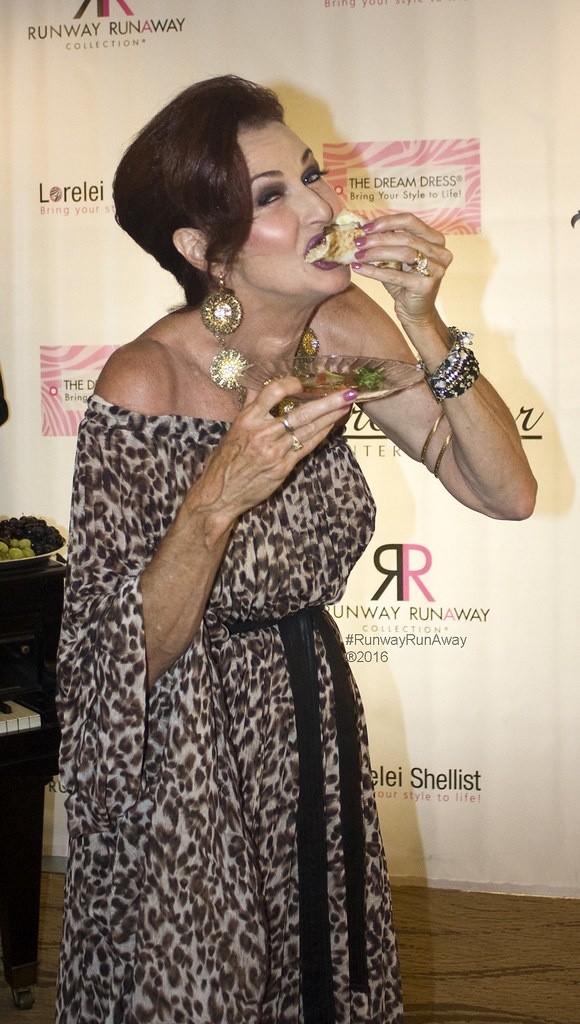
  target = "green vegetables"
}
[352,365,383,390]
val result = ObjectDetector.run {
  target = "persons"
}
[58,73,536,1024]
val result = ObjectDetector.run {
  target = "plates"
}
[236,356,425,403]
[0,539,67,572]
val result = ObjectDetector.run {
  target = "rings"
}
[280,412,294,432]
[407,250,432,277]
[291,433,303,451]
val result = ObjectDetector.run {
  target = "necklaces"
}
[202,293,320,415]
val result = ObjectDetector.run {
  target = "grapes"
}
[0,516,65,561]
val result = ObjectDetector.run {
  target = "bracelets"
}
[421,326,480,405]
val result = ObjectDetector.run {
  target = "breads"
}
[304,210,405,271]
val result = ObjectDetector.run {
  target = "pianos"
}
[0,557,67,1008]
